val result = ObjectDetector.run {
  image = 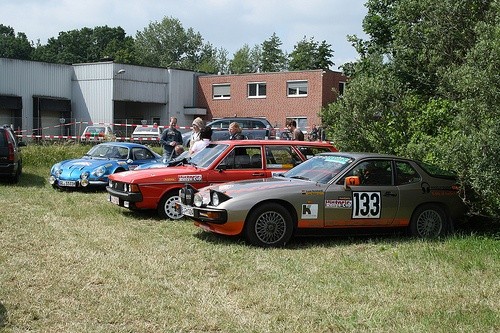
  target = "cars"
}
[274,130,293,141]
[49,140,163,191]
[132,120,163,142]
[80,124,117,145]
[134,151,193,171]
[175,151,464,248]
[106,139,339,220]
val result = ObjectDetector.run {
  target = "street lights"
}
[110,67,127,139]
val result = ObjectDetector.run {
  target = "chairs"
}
[234,154,250,169]
[250,153,262,168]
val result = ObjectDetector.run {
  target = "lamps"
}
[115,68,125,76]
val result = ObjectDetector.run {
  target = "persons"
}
[189,126,212,155]
[190,117,203,152]
[317,124,323,139]
[229,123,246,157]
[175,145,184,157]
[312,124,317,140]
[288,120,304,141]
[160,116,183,159]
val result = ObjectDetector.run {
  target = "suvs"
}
[0,122,27,184]
[178,115,277,151]
